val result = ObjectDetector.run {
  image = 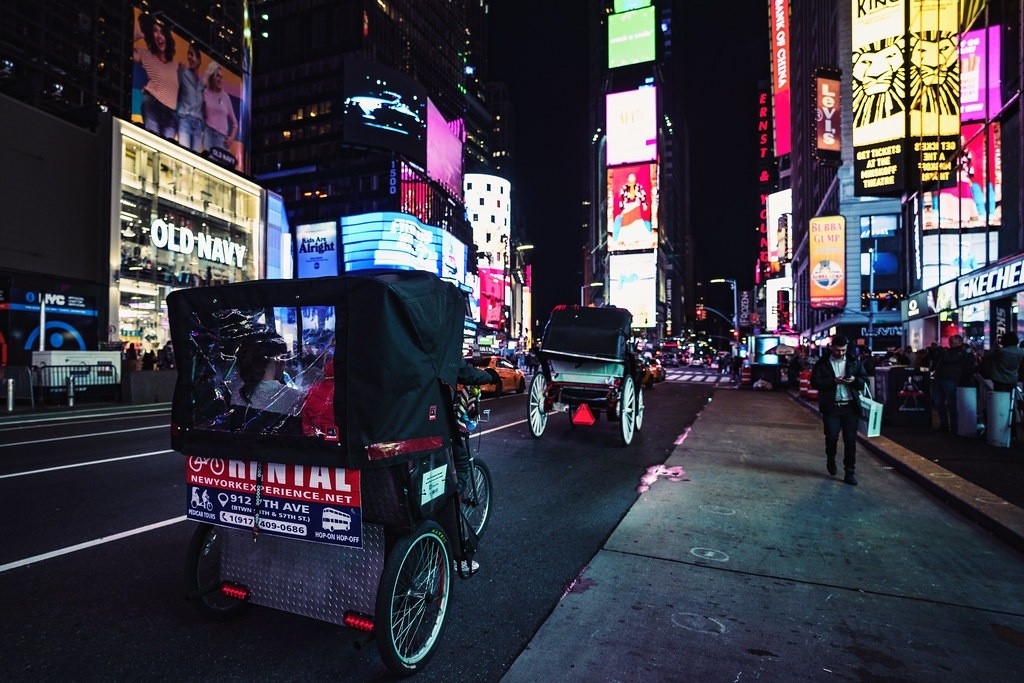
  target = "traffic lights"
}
[696,309,706,320]
[734,330,737,341]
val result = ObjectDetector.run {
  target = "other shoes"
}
[844,474,857,485]
[827,458,836,475]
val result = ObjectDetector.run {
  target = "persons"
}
[134,12,237,152]
[233,332,306,414]
[940,134,978,222]
[127,340,175,370]
[619,174,650,246]
[455,358,499,494]
[810,335,869,484]
[860,332,1024,437]
[510,351,540,375]
[789,350,808,391]
[717,355,743,381]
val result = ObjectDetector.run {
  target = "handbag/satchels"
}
[856,382,884,438]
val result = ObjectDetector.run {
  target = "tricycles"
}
[166,266,494,673]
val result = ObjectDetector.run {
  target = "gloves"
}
[484,369,501,385]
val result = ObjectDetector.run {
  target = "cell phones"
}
[843,375,851,379]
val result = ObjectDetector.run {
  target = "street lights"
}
[580,282,604,308]
[782,286,795,328]
[711,279,741,381]
[514,245,535,340]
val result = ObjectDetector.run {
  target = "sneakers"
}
[453,559,480,572]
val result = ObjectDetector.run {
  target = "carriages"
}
[525,311,648,445]
